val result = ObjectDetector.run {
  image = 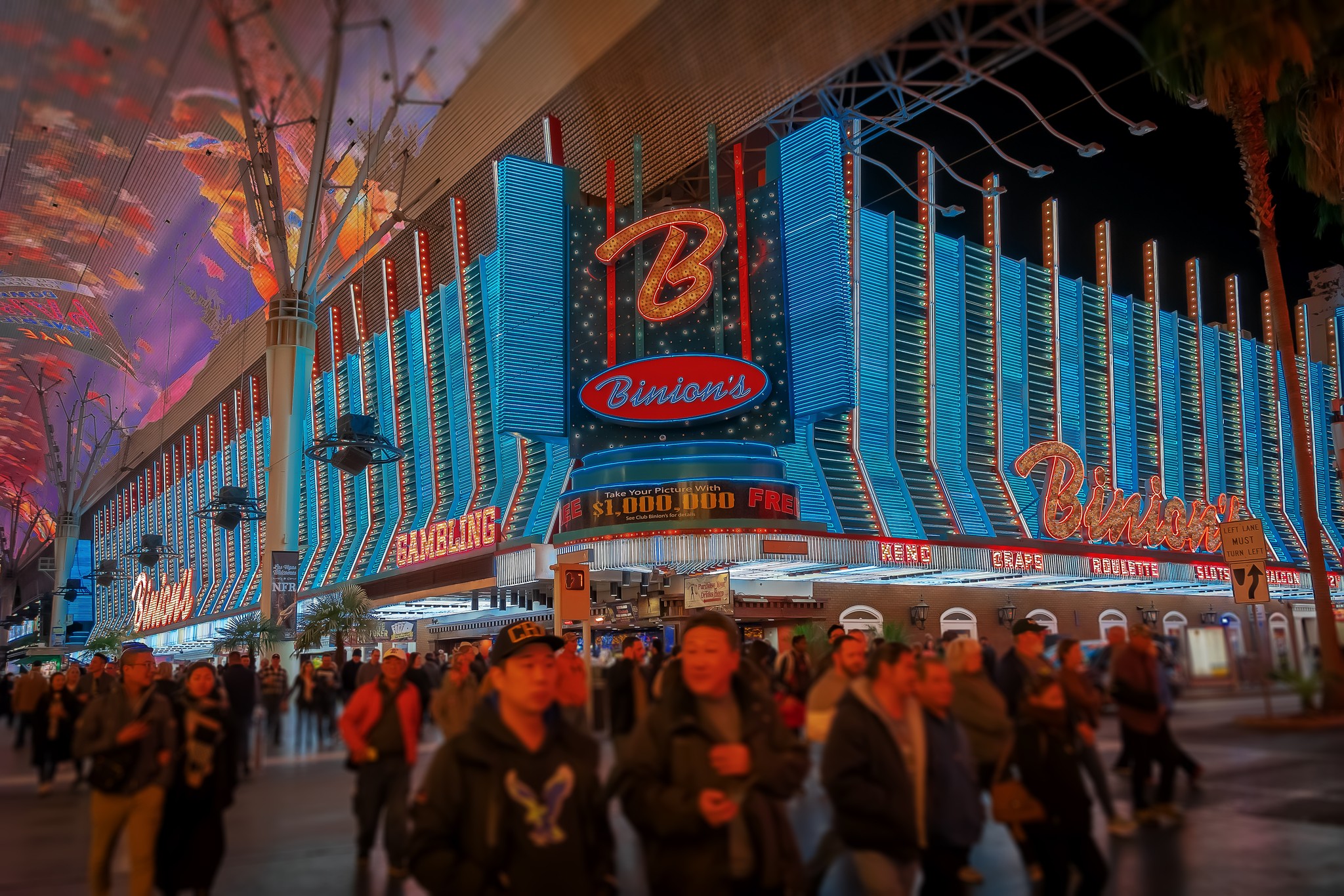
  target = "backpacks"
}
[774,651,794,676]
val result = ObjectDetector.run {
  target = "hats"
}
[272,654,280,659]
[1012,618,1048,634]
[438,649,444,654]
[32,660,44,666]
[562,632,581,641]
[382,648,407,666]
[489,619,564,664]
[459,642,472,649]
[472,641,482,646]
[937,630,959,641]
[1129,623,1164,638]
[352,648,362,657]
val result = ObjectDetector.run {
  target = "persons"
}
[0,644,290,896]
[287,611,1200,896]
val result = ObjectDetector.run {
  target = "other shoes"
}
[1133,808,1159,821]
[1154,806,1184,824]
[1188,762,1205,788]
[1109,817,1135,836]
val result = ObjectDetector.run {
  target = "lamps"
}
[910,593,930,630]
[998,595,1017,630]
[192,485,266,532]
[472,589,553,611]
[590,571,671,604]
[1137,599,1160,630]
[82,559,132,588]
[51,578,92,602]
[306,414,405,476]
[0,603,48,631]
[123,534,181,569]
[594,615,606,622]
[1200,604,1217,625]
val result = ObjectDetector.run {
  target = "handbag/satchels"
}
[346,754,361,769]
[989,734,1045,823]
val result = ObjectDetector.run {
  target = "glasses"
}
[127,663,160,672]
[830,633,844,638]
[373,653,380,657]
